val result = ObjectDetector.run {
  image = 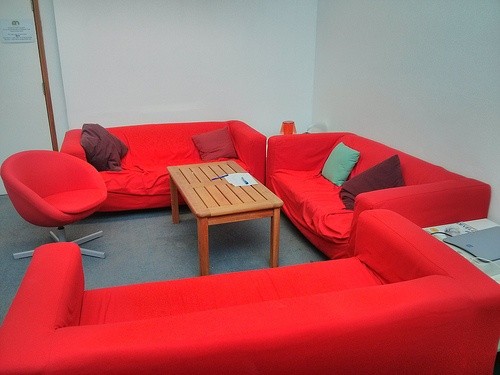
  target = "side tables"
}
[421,218,500,284]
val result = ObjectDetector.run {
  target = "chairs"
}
[0,150,105,259]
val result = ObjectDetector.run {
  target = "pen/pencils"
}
[242,177,248,184]
[212,174,228,180]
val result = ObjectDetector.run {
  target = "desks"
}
[167,159,283,276]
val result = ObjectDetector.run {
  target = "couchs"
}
[265,132,492,260]
[0,209,500,375]
[59,120,266,213]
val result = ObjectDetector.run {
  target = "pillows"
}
[193,127,239,162]
[81,124,127,171]
[339,155,403,212]
[322,142,360,186]
